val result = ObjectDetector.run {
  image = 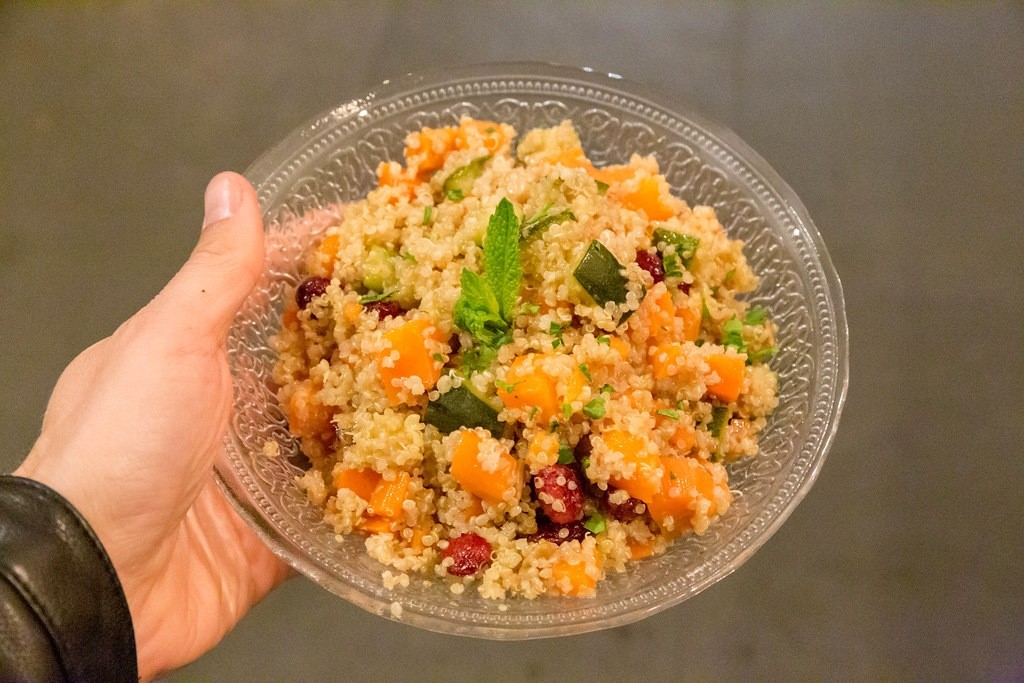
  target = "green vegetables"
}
[453,197,522,346]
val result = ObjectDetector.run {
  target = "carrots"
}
[257,116,748,597]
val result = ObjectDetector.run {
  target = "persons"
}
[0,171,368,683]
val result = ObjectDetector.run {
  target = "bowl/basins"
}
[197,55,851,638]
